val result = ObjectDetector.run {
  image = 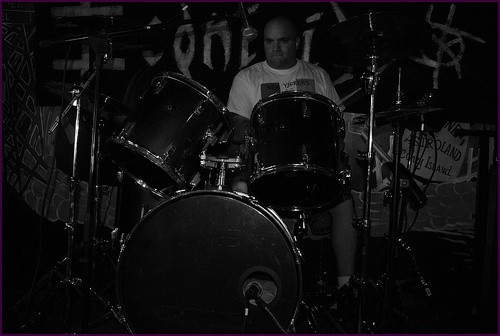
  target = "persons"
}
[225,16,363,311]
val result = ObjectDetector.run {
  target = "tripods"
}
[3,13,241,335]
[296,38,418,335]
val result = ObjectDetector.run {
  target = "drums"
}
[115,188,304,335]
[248,91,347,214]
[106,70,234,197]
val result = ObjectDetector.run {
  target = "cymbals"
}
[353,107,445,122]
[334,13,409,38]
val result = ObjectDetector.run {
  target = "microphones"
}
[238,2,257,39]
